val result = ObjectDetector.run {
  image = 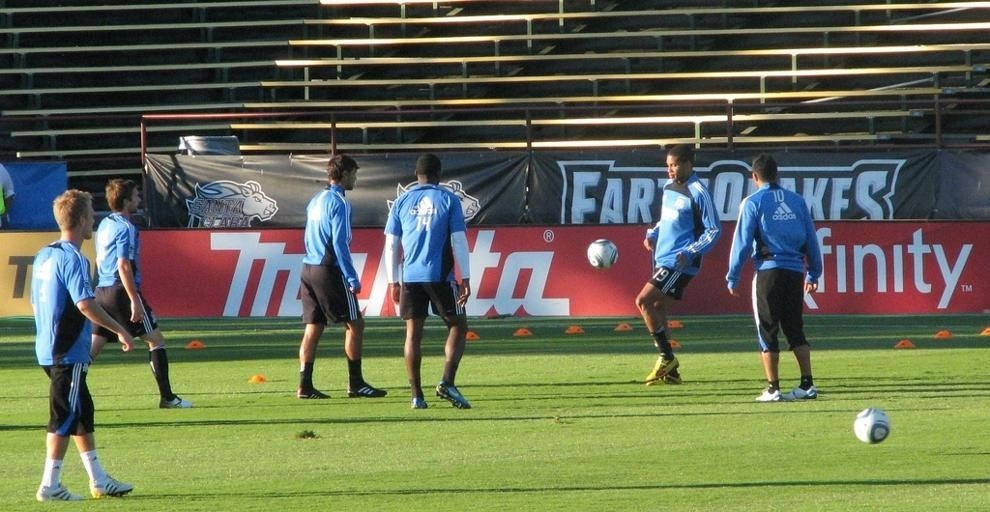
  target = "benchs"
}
[1,0,989,232]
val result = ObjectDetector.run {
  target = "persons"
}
[85,176,197,409]
[383,153,473,411]
[29,187,137,503]
[726,153,822,403]
[0,163,16,230]
[296,153,388,399]
[634,142,723,387]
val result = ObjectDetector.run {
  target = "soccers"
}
[854,409,891,443]
[587,238,616,270]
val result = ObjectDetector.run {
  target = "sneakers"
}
[755,386,783,403]
[36,481,83,500]
[160,396,192,408]
[89,474,133,496]
[298,381,471,411]
[788,384,818,402]
[646,355,681,384]
